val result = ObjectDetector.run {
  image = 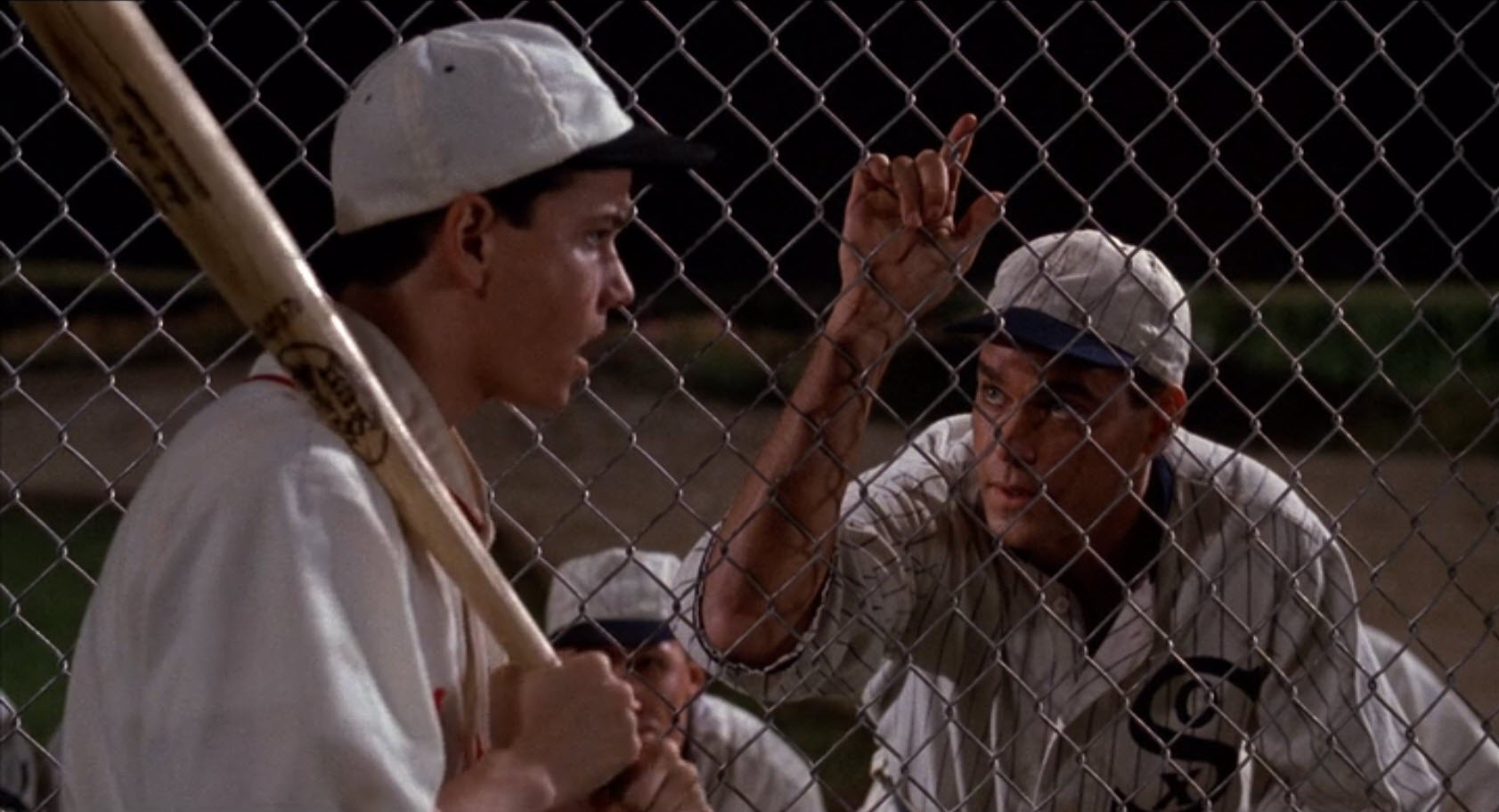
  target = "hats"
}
[936,232,1197,394]
[539,549,689,649]
[327,18,717,248]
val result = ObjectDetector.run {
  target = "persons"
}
[58,19,711,812]
[546,549,824,811]
[1356,618,1498,812]
[671,111,1399,812]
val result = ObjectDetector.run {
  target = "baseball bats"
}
[3,3,559,671]
